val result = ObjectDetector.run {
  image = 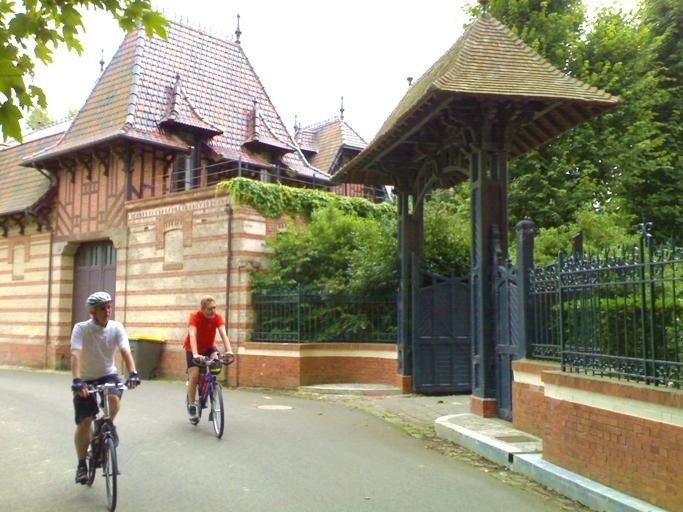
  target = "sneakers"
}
[75,465,88,485]
[111,424,119,447]
[187,403,199,421]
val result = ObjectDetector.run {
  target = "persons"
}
[183,294,234,419]
[68,291,141,483]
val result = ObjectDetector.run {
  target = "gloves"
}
[71,377,88,392]
[124,371,142,387]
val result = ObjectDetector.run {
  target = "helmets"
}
[85,291,113,312]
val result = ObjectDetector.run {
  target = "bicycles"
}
[73,379,129,512]
[187,355,228,439]
